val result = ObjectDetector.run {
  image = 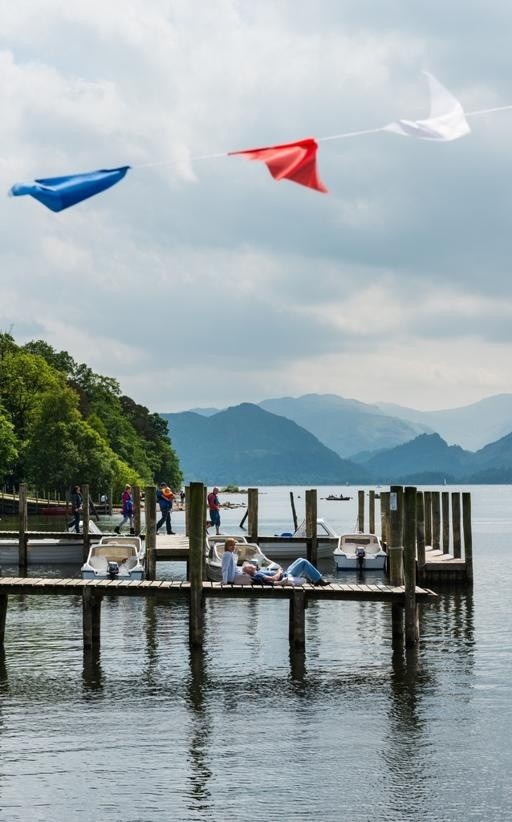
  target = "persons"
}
[156,483,176,534]
[242,558,332,586]
[179,490,186,506]
[66,485,83,533]
[207,487,222,535]
[219,538,288,586]
[163,487,176,503]
[114,484,135,534]
[101,493,108,504]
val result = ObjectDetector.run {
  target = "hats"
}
[225,538,238,546]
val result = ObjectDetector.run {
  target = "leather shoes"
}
[311,579,331,587]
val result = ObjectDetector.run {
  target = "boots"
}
[114,525,121,534]
[129,527,136,535]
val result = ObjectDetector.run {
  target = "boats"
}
[0,520,105,564]
[206,542,283,581]
[203,535,247,557]
[98,536,146,568]
[367,489,382,499]
[332,530,387,572]
[324,492,351,502]
[259,518,340,560]
[79,543,144,579]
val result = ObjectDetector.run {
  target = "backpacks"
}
[212,495,221,507]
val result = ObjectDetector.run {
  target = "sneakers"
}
[166,532,176,535]
[156,531,159,535]
